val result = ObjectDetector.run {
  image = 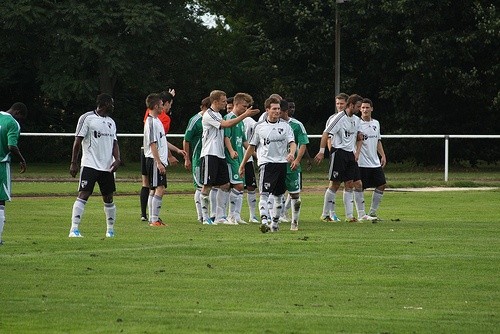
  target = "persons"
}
[143,94,188,227]
[182,90,306,233]
[314,93,387,222]
[139,88,175,221]
[68,94,121,239]
[0,101,27,246]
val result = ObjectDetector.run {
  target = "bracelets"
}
[70,161,79,164]
[319,147,325,154]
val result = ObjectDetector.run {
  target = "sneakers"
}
[320,215,334,222]
[69,232,84,238]
[141,217,148,221]
[148,220,168,226]
[330,216,341,222]
[358,215,377,222]
[345,217,358,222]
[367,214,384,221]
[248,217,260,223]
[268,214,299,231]
[158,218,163,221]
[260,223,278,233]
[106,230,115,237]
[197,217,246,225]
